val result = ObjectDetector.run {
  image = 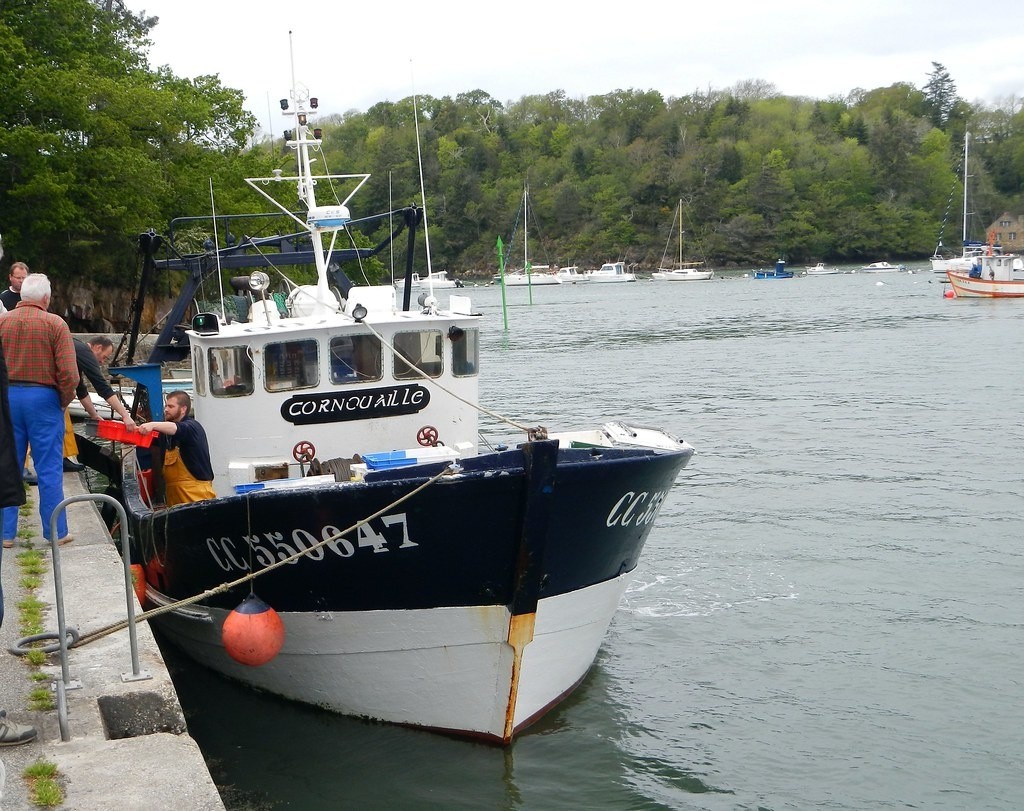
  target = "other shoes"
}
[3,540,13,548]
[63,457,85,473]
[23,467,39,485]
[49,532,73,548]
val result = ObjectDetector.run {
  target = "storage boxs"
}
[85,417,159,448]
[362,445,460,472]
[233,473,335,494]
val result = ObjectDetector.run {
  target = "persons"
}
[140,390,216,507]
[0,234,137,746]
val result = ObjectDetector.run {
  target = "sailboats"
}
[493,187,564,285]
[927,130,1024,275]
[651,198,714,283]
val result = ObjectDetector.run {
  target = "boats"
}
[61,368,196,420]
[416,269,466,290]
[587,261,637,283]
[805,261,840,276]
[861,262,908,274]
[72,31,692,744]
[942,231,1024,298]
[936,275,950,284]
[556,265,590,283]
[751,258,795,279]
[394,272,423,289]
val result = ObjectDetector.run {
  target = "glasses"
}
[11,274,26,281]
